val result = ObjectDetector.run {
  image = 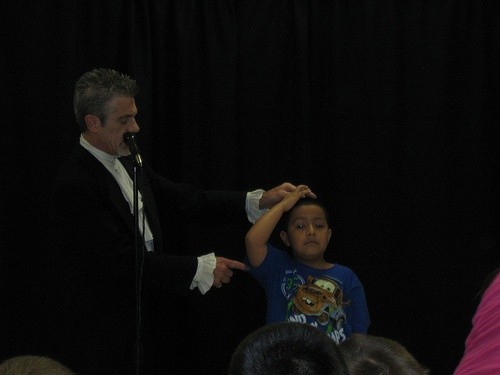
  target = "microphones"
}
[122,131,143,168]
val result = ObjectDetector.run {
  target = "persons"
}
[0,268,500,375]
[245,185,371,346]
[47,67,316,375]
